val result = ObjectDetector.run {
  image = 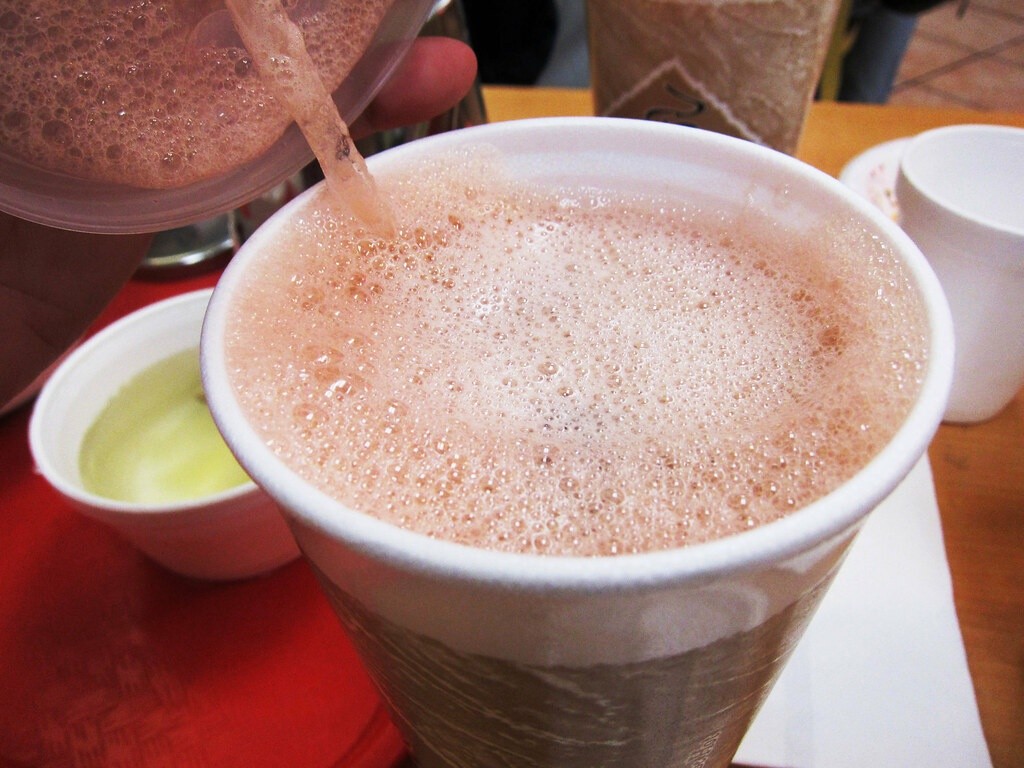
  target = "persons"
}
[1,38,478,410]
[813,0,951,108]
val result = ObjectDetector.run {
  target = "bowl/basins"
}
[29,285,301,581]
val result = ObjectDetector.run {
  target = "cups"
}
[588,0,840,158]
[896,124,1024,423]
[199,116,953,768]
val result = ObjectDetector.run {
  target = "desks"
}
[0,82,1024,768]
